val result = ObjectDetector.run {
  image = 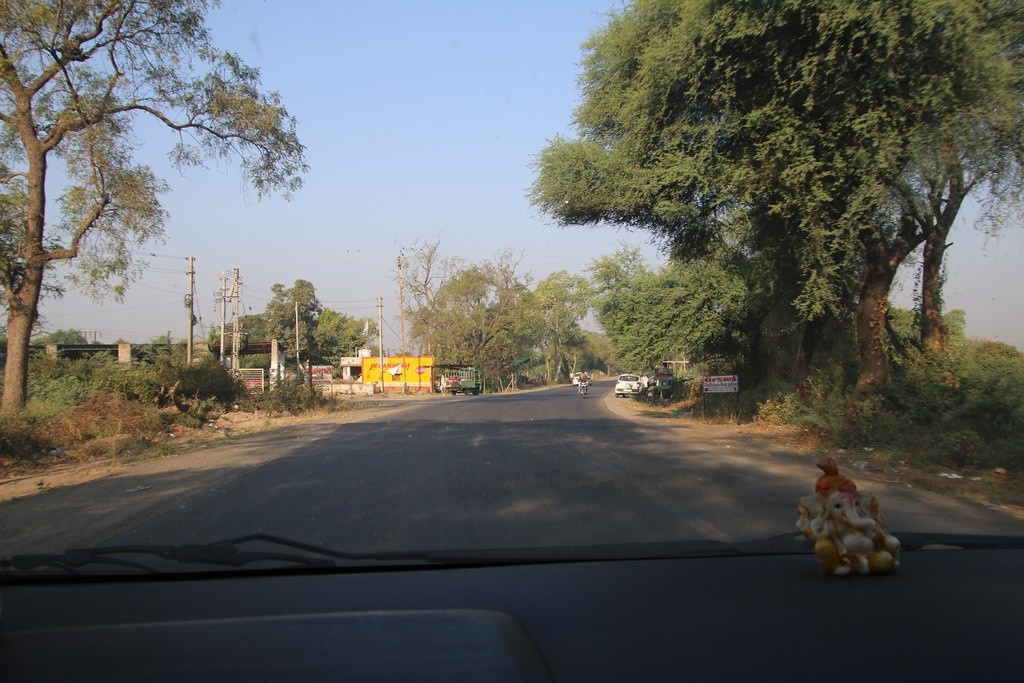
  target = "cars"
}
[572,372,592,387]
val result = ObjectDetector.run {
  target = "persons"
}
[578,371,590,392]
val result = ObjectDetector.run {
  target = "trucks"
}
[442,367,483,396]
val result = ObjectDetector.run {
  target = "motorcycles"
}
[577,378,591,399]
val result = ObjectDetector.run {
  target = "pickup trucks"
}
[614,374,642,398]
